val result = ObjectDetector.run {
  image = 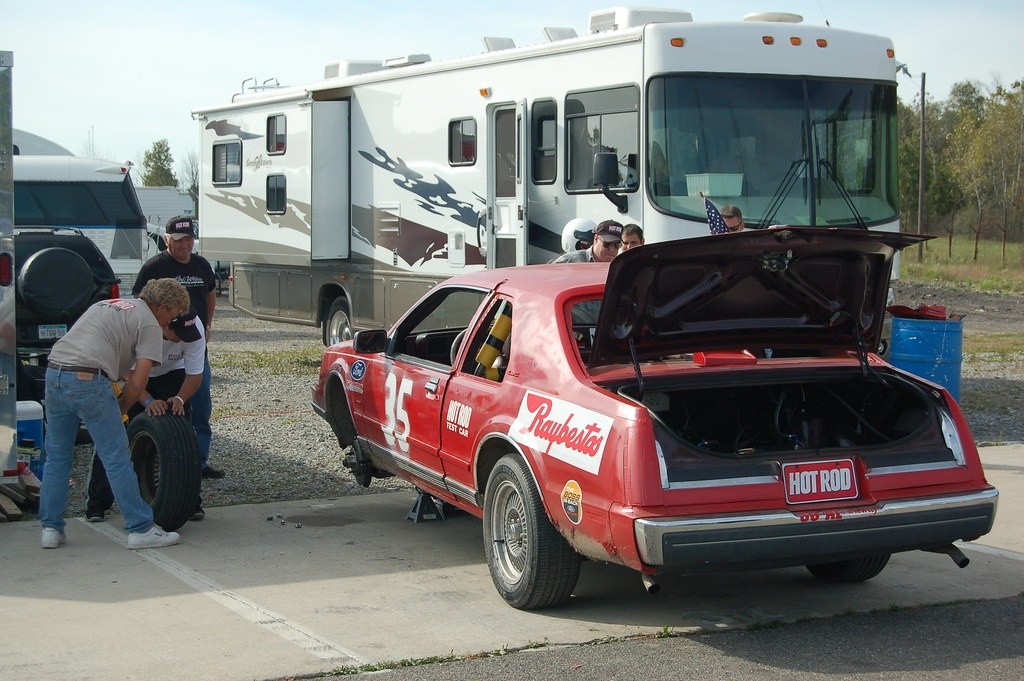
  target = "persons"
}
[621,224,645,251]
[552,220,624,324]
[132,217,226,480]
[721,205,744,232]
[40,279,190,550]
[84,306,212,522]
[547,217,599,264]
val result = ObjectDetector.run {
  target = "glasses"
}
[599,238,623,249]
[728,222,742,232]
[167,306,178,322]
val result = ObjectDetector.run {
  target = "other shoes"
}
[201,465,226,479]
[85,509,104,522]
[189,506,205,519]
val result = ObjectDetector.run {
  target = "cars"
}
[308,224,999,610]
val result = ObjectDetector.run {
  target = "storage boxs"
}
[685,172,744,198]
[16,400,44,449]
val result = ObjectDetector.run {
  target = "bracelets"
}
[175,396,184,405]
[143,397,153,406]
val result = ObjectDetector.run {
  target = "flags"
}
[703,197,728,235]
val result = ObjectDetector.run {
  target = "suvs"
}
[12,154,167,444]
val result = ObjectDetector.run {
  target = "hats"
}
[171,305,202,343]
[597,219,623,242]
[166,215,197,240]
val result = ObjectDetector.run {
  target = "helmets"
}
[561,218,597,252]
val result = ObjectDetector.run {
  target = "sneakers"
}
[128,524,180,549]
[41,527,66,548]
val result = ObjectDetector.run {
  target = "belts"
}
[48,362,107,376]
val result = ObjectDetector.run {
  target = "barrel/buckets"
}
[888,317,963,409]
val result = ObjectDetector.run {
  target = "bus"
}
[190,6,914,356]
